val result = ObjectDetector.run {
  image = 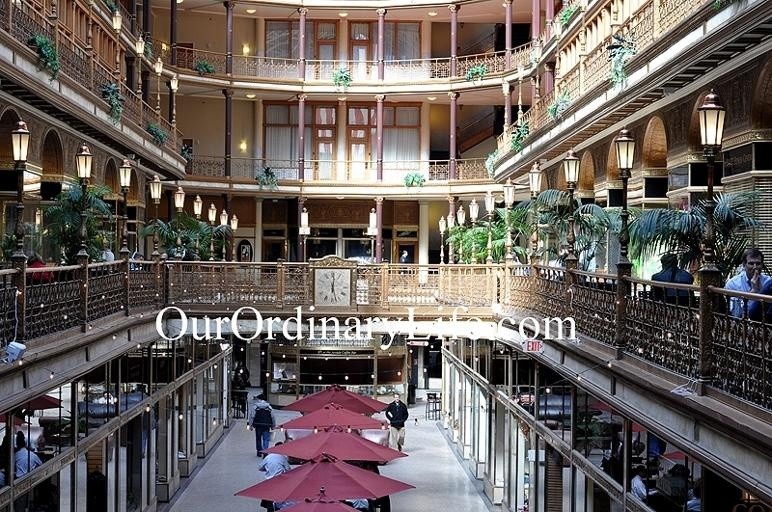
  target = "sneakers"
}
[397,442,402,451]
[257,450,268,460]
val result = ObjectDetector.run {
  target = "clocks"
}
[312,266,352,308]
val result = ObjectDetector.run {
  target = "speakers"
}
[6,342,26,361]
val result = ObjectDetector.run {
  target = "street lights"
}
[695,87,728,270]
[173,184,239,260]
[148,172,167,261]
[438,176,519,264]
[73,140,91,259]
[528,161,543,257]
[563,148,582,262]
[116,157,135,260]
[612,127,639,263]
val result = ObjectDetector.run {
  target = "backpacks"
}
[252,406,273,429]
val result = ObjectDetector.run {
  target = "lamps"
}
[74,142,94,257]
[438,149,581,296]
[109,9,179,126]
[611,129,638,363]
[695,84,728,392]
[117,158,239,257]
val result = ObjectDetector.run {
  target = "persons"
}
[14,431,43,479]
[248,393,277,459]
[25,252,55,283]
[385,392,409,452]
[400,250,409,274]
[722,249,772,322]
[630,464,661,502]
[256,441,293,512]
[103,245,115,261]
[96,391,118,404]
[651,253,694,307]
[681,484,702,512]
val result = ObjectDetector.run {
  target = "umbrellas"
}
[274,485,363,512]
[271,402,391,432]
[231,451,419,501]
[277,383,393,415]
[259,423,409,462]
